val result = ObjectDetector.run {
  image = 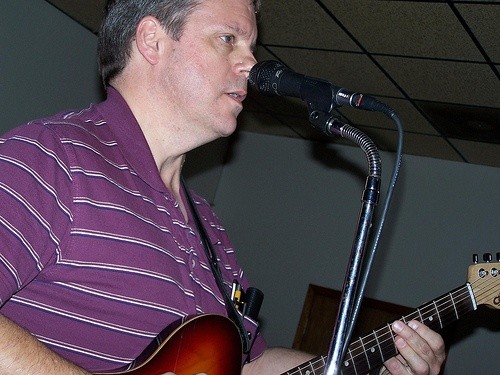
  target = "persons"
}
[0,0,447,375]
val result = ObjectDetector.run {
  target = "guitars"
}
[91,252,500,375]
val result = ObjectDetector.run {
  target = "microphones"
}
[249,60,396,118]
